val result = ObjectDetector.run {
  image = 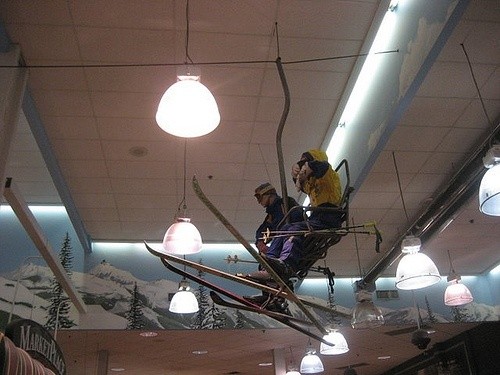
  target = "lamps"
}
[286,346,301,375]
[300,329,324,373]
[170,256,201,313]
[351,217,384,330]
[155,0,221,139]
[320,260,350,356]
[164,139,203,255]
[444,251,472,306]
[393,153,441,290]
[461,43,500,217]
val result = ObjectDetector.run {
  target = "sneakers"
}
[243,295,250,301]
[250,295,292,316]
[240,269,282,284]
[260,251,298,291]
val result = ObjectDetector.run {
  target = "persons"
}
[243,149,341,284]
[243,182,308,317]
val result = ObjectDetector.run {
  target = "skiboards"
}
[159,257,336,347]
[144,176,352,334]
